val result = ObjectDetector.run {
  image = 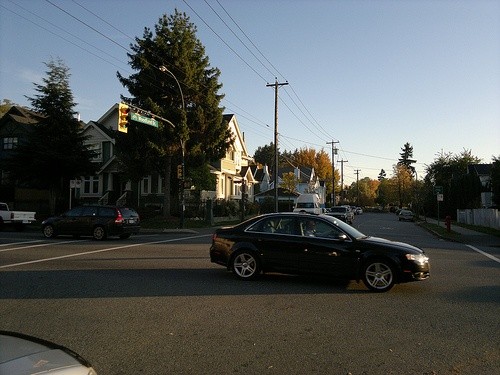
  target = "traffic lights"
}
[117,103,130,134]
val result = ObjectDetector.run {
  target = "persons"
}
[305,221,316,236]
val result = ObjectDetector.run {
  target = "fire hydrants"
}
[445,217,451,233]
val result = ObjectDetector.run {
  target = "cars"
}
[210,213,430,291]
[41,205,141,241]
[325,205,363,224]
[390,206,415,221]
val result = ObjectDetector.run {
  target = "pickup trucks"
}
[0,203,36,230]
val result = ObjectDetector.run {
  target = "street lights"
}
[159,65,185,229]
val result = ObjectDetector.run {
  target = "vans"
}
[293,194,322,215]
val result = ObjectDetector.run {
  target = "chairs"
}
[264,221,276,233]
